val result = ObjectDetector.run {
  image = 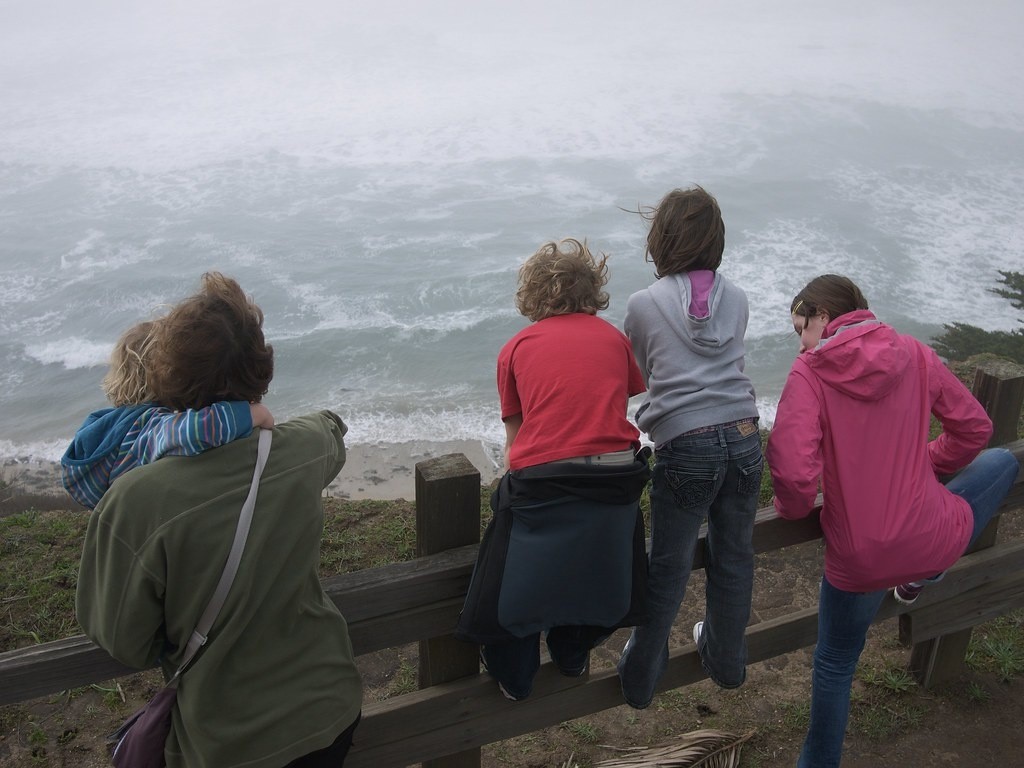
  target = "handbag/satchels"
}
[106,684,177,768]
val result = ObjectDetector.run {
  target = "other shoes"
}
[693,621,704,643]
[623,639,630,652]
[479,645,531,700]
[894,582,923,605]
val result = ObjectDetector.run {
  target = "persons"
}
[615,188,763,711]
[62,270,362,768]
[474,236,650,709]
[763,274,1020,768]
